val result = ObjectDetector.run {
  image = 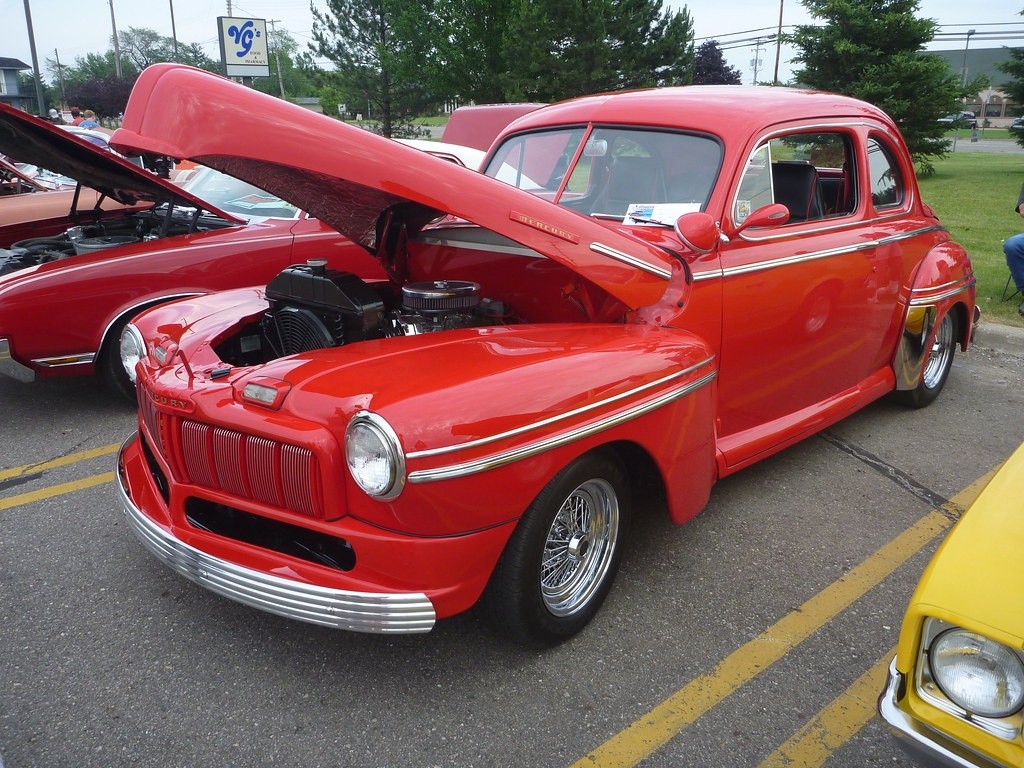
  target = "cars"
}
[108,62,982,648]
[936,111,977,130]
[878,440,1024,768]
[0,101,584,411]
[0,125,199,249]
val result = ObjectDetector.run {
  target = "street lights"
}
[952,29,975,152]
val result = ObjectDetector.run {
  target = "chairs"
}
[586,154,667,214]
[752,165,827,225]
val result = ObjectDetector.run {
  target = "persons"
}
[1001,178,1024,318]
[48,106,100,128]
